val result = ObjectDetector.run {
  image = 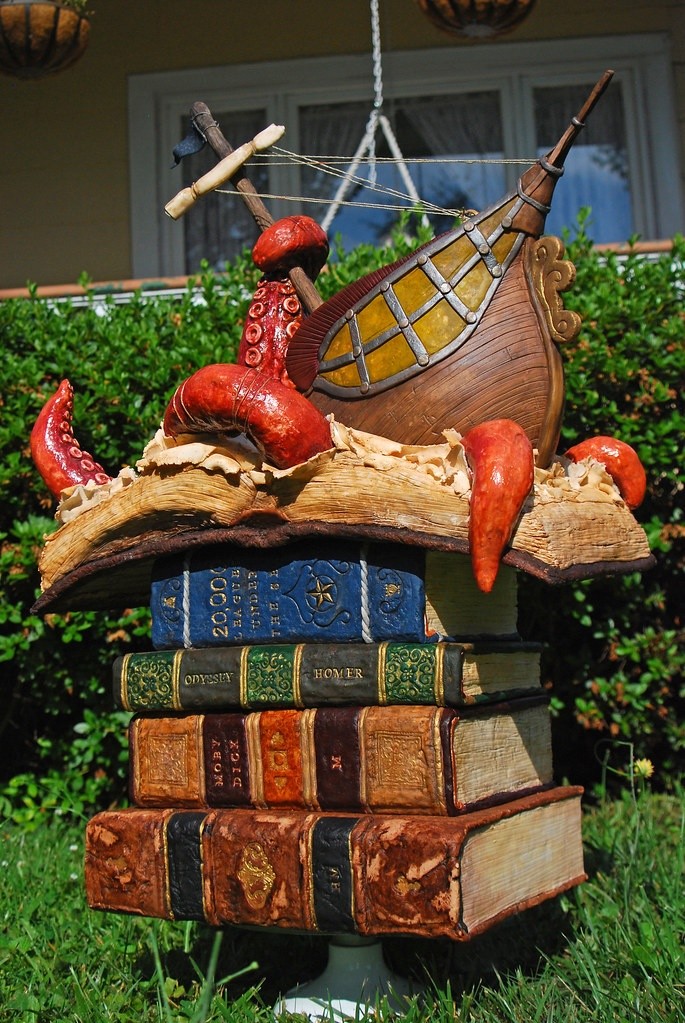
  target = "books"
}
[41,448,656,941]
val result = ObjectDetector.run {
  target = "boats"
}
[162,69,618,471]
